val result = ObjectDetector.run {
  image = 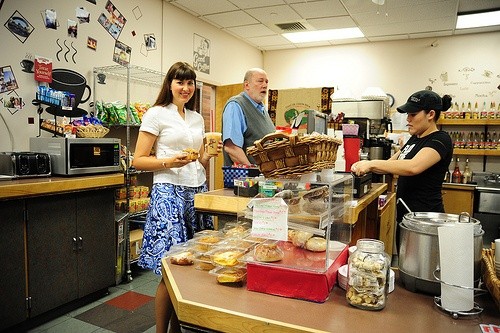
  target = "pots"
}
[398,211,484,295]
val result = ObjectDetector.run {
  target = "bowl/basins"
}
[170,222,264,285]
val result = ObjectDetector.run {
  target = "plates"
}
[336,263,394,295]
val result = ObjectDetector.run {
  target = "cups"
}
[205,131,222,155]
[327,129,348,172]
[494,239,500,279]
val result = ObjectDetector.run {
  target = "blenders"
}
[328,96,392,172]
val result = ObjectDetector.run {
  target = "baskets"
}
[245,132,342,181]
[480,248,500,302]
[77,124,109,138]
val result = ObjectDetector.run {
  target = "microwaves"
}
[30,136,121,176]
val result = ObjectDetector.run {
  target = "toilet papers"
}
[438,221,476,313]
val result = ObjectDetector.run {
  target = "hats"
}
[396,90,442,113]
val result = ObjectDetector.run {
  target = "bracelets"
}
[161,158,166,168]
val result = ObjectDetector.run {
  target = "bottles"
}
[442,168,450,183]
[449,131,500,149]
[345,238,389,310]
[452,158,461,183]
[440,100,500,119]
[462,157,472,183]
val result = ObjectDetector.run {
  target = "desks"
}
[194,183,388,230]
[154,255,500,333]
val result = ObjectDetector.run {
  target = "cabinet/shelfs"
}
[437,118,500,172]
[0,185,116,333]
[93,63,203,283]
[380,183,474,258]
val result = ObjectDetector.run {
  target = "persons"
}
[351,89,454,266]
[68,26,75,37]
[133,62,223,333]
[220,67,276,167]
[148,36,155,48]
[103,3,125,27]
[5,96,20,108]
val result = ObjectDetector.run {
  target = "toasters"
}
[0,151,51,180]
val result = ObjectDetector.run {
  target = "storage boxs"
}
[238,172,355,303]
[221,167,260,188]
[129,229,144,259]
[116,185,150,214]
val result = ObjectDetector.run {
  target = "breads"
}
[169,224,248,283]
[255,243,284,262]
[285,229,327,251]
[182,147,198,160]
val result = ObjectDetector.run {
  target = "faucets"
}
[381,117,388,132]
[388,119,392,133]
